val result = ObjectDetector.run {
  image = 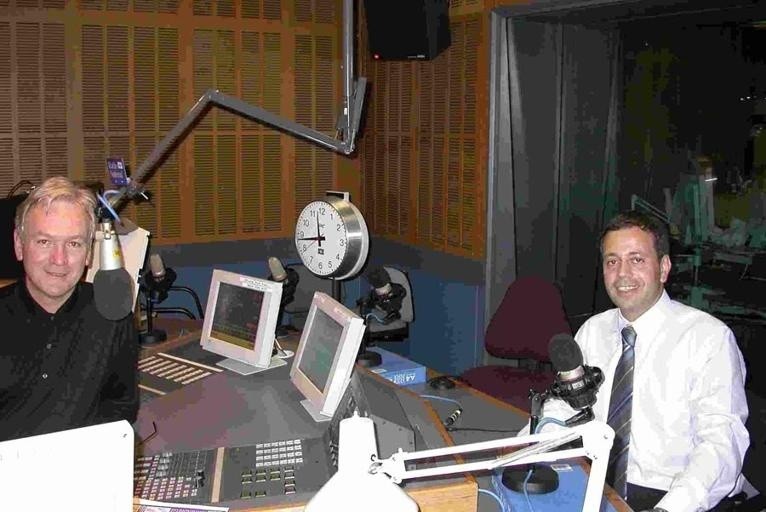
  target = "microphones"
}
[150,253,166,282]
[268,257,290,287]
[93,217,134,321]
[548,334,597,411]
[368,265,402,313]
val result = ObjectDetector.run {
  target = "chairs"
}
[740,386,766,512]
[455,279,575,415]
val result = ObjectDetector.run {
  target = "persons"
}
[0,173,144,444]
[495,207,766,512]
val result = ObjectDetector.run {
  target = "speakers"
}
[363,0,451,62]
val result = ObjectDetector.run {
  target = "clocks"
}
[295,191,369,282]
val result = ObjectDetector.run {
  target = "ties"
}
[596,326,637,502]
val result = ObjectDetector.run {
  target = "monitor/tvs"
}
[289,290,367,423]
[199,269,288,376]
[85,212,151,312]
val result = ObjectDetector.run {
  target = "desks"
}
[134,315,635,512]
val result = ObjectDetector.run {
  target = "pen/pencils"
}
[446,409,462,424]
